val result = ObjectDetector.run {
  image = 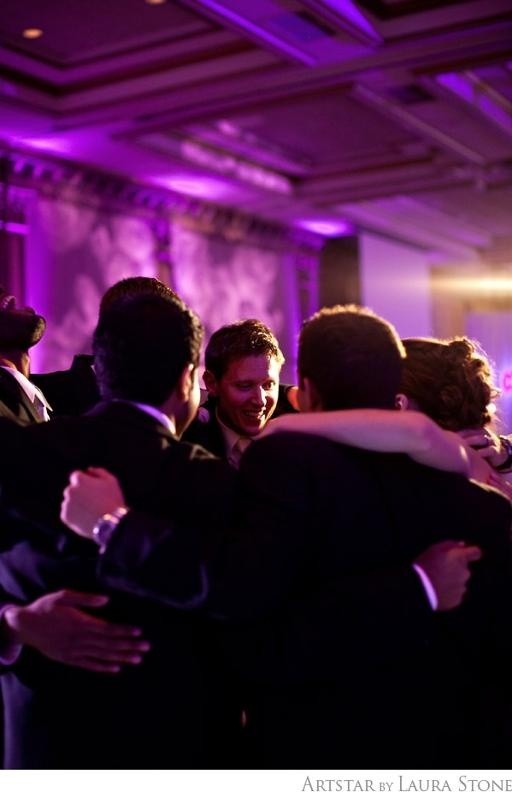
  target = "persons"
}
[30,276,171,412]
[0,588,150,676]
[251,337,512,504]
[455,426,512,470]
[60,305,512,770]
[0,291,482,768]
[182,318,296,471]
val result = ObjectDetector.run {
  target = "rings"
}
[481,435,490,442]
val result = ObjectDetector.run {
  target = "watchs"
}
[91,506,126,547]
